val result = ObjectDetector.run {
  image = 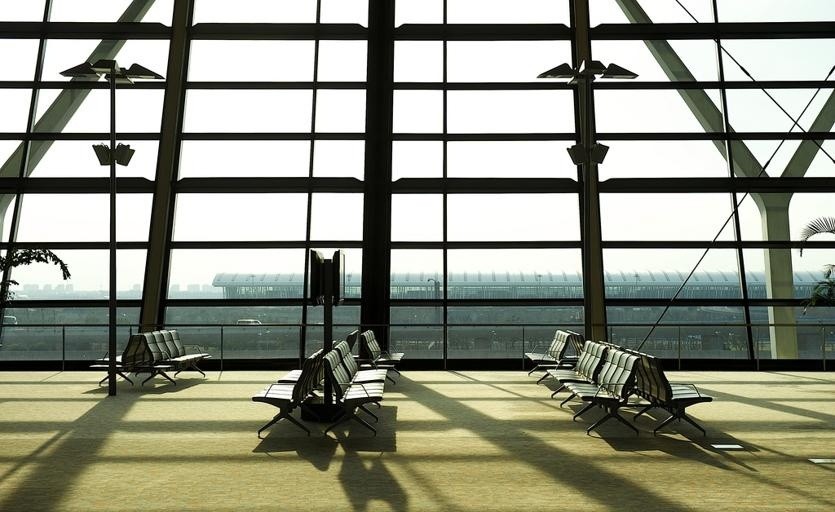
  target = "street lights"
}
[427,278,438,329]
[537,61,640,343]
[58,59,165,398]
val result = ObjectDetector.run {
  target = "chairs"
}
[248,326,406,440]
[522,322,717,437]
[87,328,215,390]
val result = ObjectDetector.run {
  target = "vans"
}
[2,315,17,325]
[236,319,262,325]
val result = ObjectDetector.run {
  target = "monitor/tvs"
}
[333,250,345,306]
[310,249,325,307]
